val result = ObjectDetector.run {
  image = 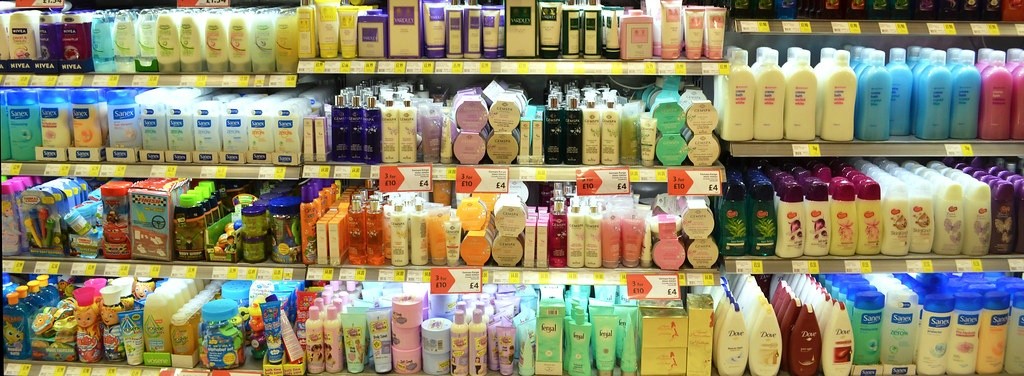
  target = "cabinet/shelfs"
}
[722,18,1024,376]
[295,56,731,376]
[0,71,315,376]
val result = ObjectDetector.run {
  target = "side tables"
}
[532,119,543,162]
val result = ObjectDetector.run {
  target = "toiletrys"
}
[0,0,1022,376]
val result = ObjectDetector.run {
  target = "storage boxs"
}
[262,350,286,376]
[686,292,714,376]
[203,213,242,263]
[537,222,548,268]
[637,306,688,376]
[315,116,329,162]
[523,223,535,268]
[282,351,307,376]
[637,298,684,310]
[316,185,366,266]
[127,186,176,262]
[170,349,199,368]
[519,118,530,163]
[302,116,314,162]
[143,351,171,367]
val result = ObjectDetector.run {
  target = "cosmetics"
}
[0,0,1024,376]
